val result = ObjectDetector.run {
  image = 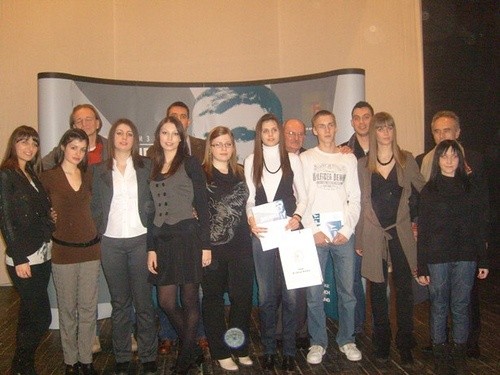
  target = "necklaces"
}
[262,158,281,174]
[377,153,395,166]
[163,160,172,170]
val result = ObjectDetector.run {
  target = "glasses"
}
[211,141,234,149]
[283,131,308,138]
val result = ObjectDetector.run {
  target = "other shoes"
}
[262,353,275,368]
[80,361,98,374]
[172,335,207,375]
[65,361,81,375]
[465,333,482,359]
[142,362,160,374]
[281,357,293,370]
[114,363,132,374]
[229,347,253,366]
[400,346,414,360]
[372,337,388,359]
[214,356,239,370]
[91,335,102,355]
[131,332,138,351]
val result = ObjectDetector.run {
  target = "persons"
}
[409,111,489,375]
[276,110,362,364]
[0,125,57,375]
[35,104,136,375]
[243,113,308,370]
[200,127,254,370]
[146,102,206,375]
[337,101,428,365]
[89,119,159,375]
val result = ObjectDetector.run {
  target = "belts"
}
[51,235,103,248]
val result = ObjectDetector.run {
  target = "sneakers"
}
[306,345,326,364]
[339,342,363,361]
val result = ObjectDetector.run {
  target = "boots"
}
[451,342,471,374]
[431,338,450,374]
